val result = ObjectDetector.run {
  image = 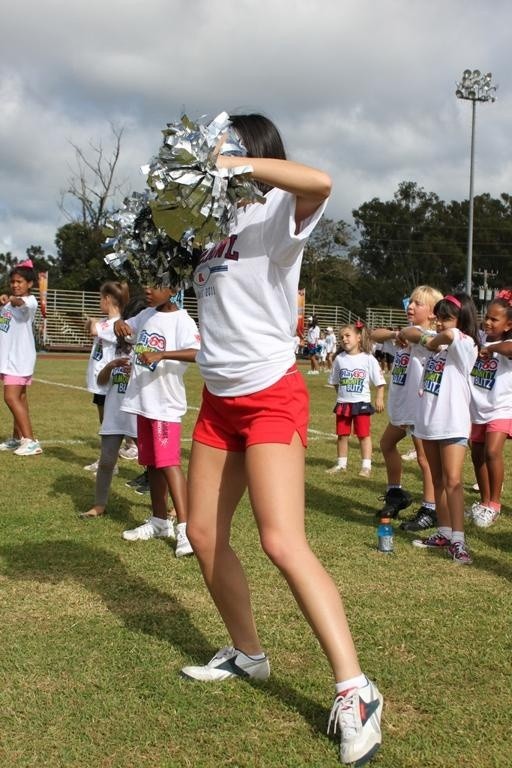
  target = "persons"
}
[306,313,322,376]
[324,321,388,478]
[112,280,195,559]
[79,298,181,518]
[374,342,385,374]
[81,279,140,477]
[374,282,444,534]
[171,108,390,767]
[467,287,512,530]
[325,325,338,373]
[381,326,395,374]
[0,257,46,458]
[398,290,483,567]
[318,329,329,373]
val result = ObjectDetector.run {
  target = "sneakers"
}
[118,445,141,461]
[326,678,385,767]
[410,532,452,551]
[326,463,346,475]
[0,439,21,451]
[449,539,472,564]
[398,504,440,532]
[173,522,195,557]
[180,647,272,685]
[125,473,146,487]
[466,501,483,516]
[307,369,320,375]
[13,439,44,456]
[401,450,416,463]
[134,480,154,496]
[121,515,174,543]
[83,460,119,474]
[357,465,372,478]
[475,504,501,527]
[376,487,413,519]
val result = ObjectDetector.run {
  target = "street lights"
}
[455,68,498,298]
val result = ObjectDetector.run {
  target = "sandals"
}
[78,509,107,519]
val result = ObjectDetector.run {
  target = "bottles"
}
[378,519,394,552]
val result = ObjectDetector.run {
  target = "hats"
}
[307,316,313,321]
